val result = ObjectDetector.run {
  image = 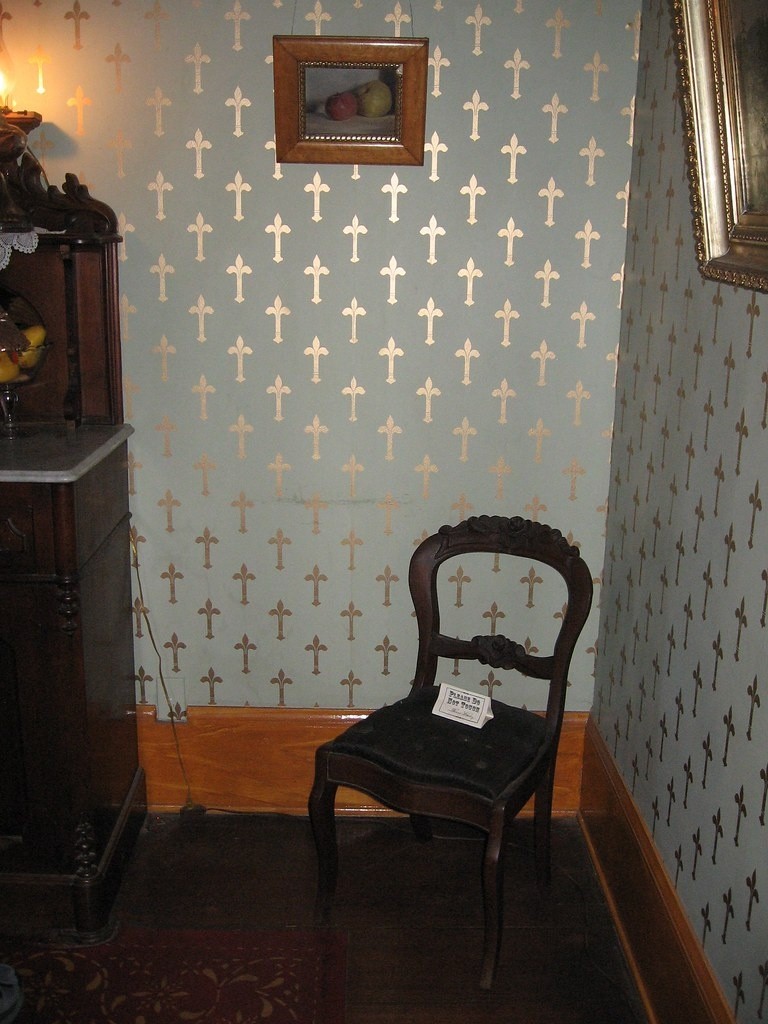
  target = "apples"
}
[326,79,394,120]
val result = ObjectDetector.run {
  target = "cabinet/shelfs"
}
[1,110,150,947]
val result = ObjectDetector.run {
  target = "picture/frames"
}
[272,35,430,166]
[671,0,768,293]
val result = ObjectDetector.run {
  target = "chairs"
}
[309,515,594,996]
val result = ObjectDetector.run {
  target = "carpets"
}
[0,928,348,1024]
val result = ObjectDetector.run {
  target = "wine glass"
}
[0,342,53,441]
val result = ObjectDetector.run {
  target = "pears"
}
[0,323,48,384]
